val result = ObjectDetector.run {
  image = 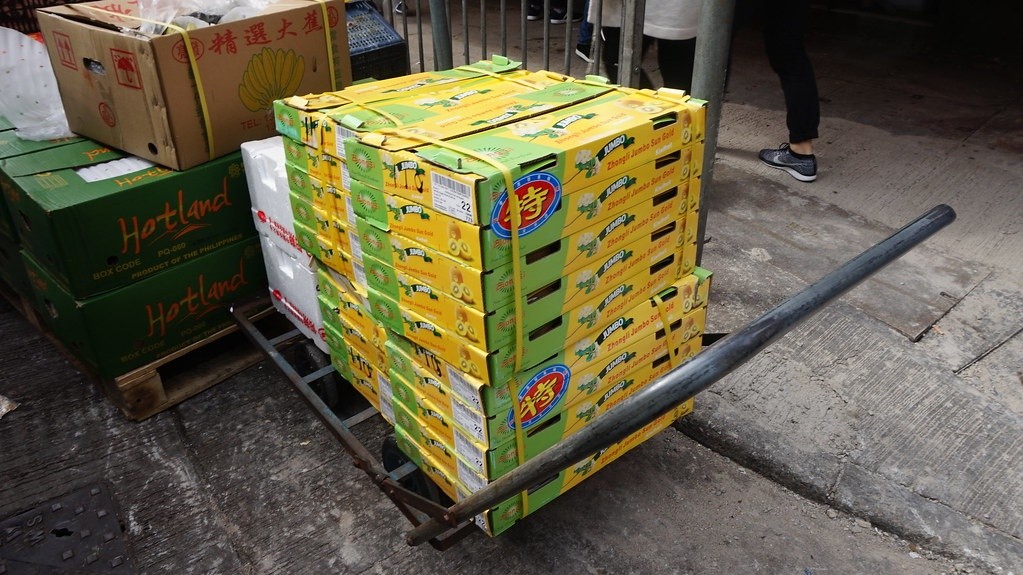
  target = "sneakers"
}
[760,142,817,182]
[527,6,544,20]
[550,9,583,23]
[601,28,607,41]
[575,44,595,64]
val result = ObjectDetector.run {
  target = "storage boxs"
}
[0,0,709,538]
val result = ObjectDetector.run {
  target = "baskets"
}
[346,0,411,81]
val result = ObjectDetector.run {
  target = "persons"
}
[526,0,593,63]
[392,0,409,14]
[586,0,821,183]
